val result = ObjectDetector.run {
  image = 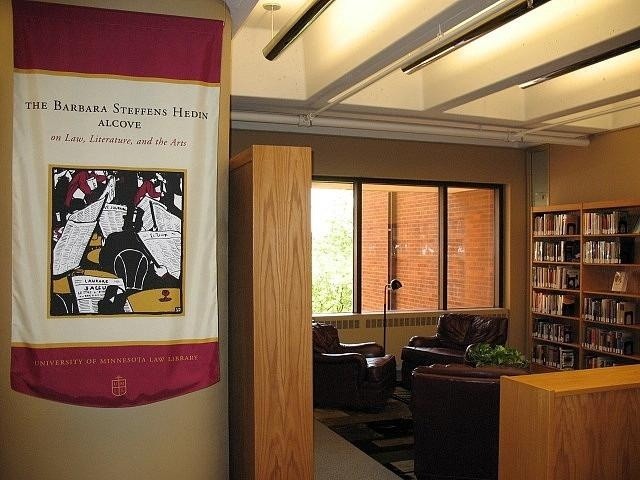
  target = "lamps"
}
[400,312,508,390]
[312,323,396,410]
[410,364,529,480]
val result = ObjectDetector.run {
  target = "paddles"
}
[313,386,416,480]
[314,385,416,480]
[314,369,417,480]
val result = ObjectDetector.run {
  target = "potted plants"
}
[559,347,576,371]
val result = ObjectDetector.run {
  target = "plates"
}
[498,364,640,480]
[527,204,581,371]
[581,200,640,370]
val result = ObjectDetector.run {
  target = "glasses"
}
[383,279,402,355]
[401,0,550,75]
[261,0,335,61]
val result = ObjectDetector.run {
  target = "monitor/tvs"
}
[531,210,640,371]
